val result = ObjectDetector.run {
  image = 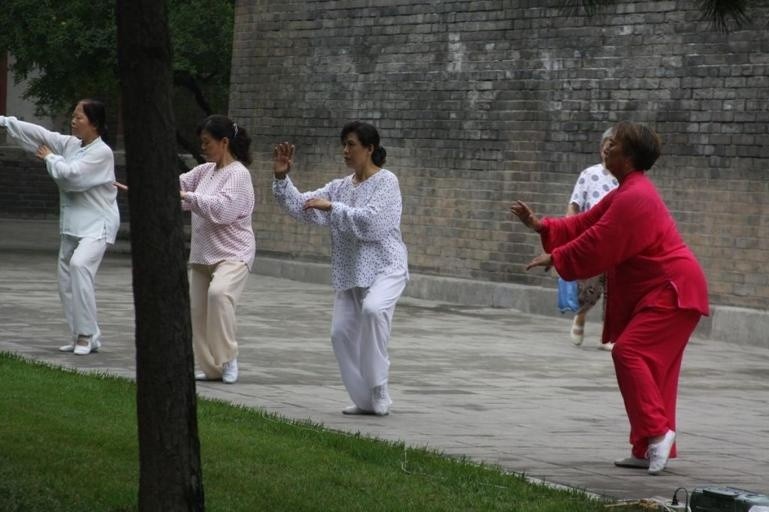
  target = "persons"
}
[114,114,255,385]
[510,122,710,474]
[272,121,409,416]
[0,100,120,354]
[564,125,622,352]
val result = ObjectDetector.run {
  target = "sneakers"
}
[61,341,101,353]
[75,334,94,356]
[600,339,618,351]
[571,315,586,343]
[194,370,210,380]
[646,429,675,474]
[222,357,238,384]
[615,454,651,468]
[371,381,388,414]
[343,404,370,414]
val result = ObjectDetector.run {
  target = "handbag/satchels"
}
[557,275,579,315]
